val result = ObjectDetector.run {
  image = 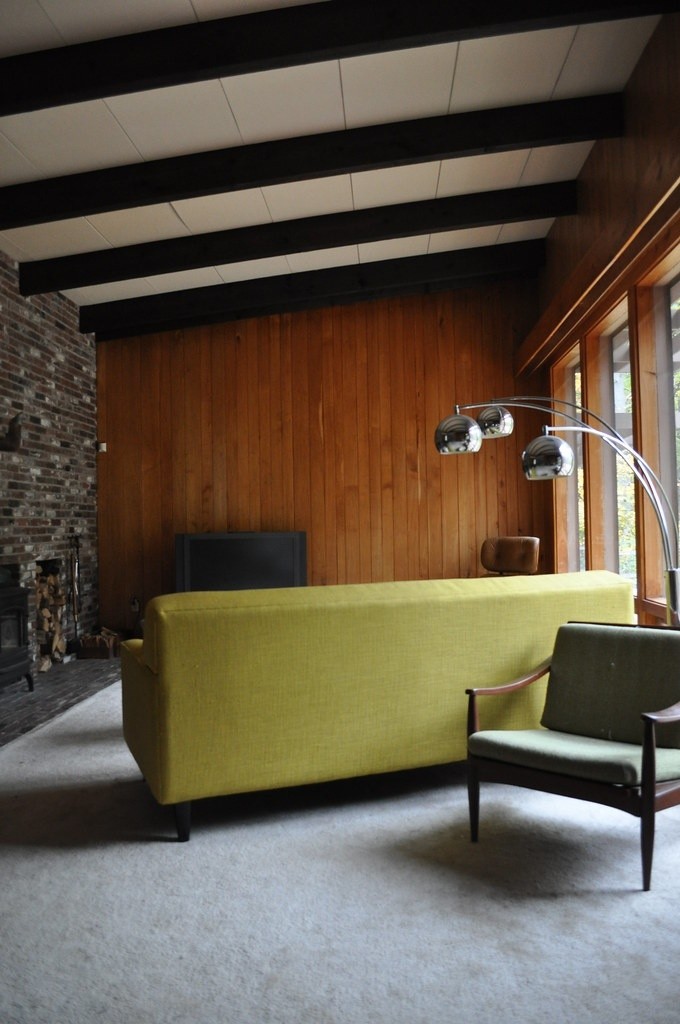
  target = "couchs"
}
[119,570,636,843]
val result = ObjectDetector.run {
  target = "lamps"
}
[433,395,680,626]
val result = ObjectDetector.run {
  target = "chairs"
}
[464,620,680,891]
[480,536,540,577]
